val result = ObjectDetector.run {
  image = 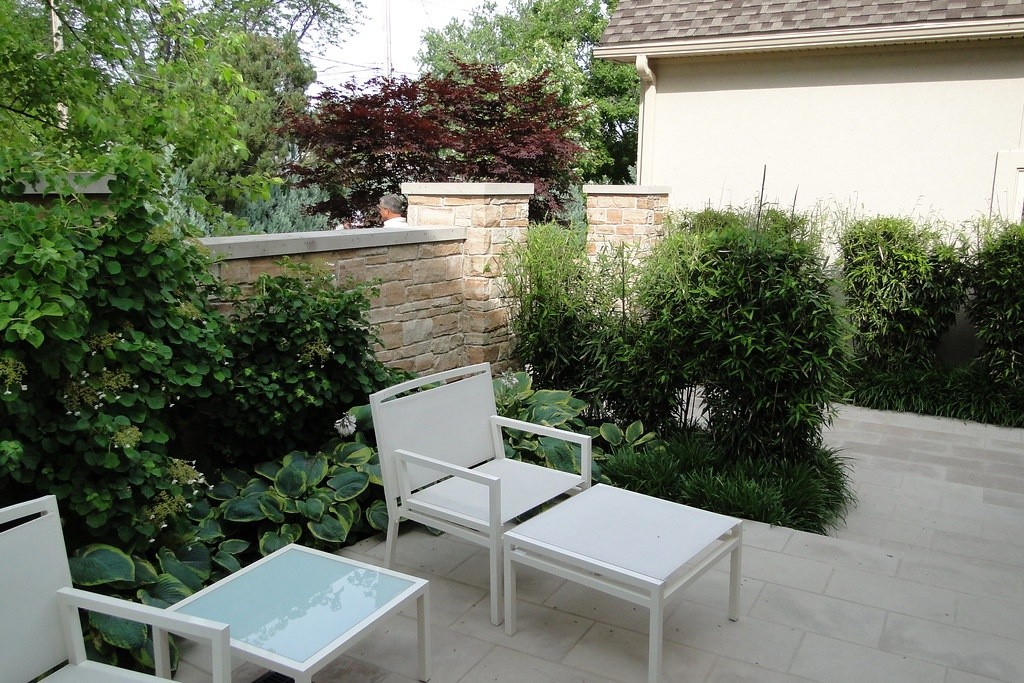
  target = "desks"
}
[503,483,744,683]
[163,543,432,683]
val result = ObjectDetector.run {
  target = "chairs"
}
[0,495,228,683]
[365,362,593,626]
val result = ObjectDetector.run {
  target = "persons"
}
[379,195,409,229]
